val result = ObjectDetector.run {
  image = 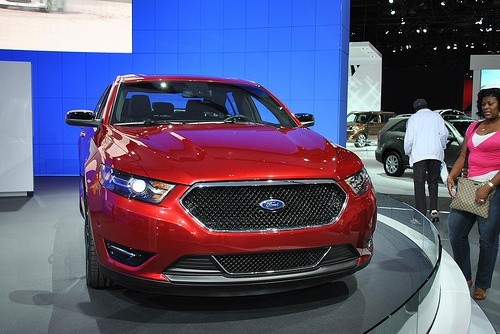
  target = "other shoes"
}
[466,279,472,289]
[409,218,426,226]
[430,210,440,223]
[472,286,486,300]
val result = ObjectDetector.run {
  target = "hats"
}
[413,99,428,111]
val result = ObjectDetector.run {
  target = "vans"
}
[346,111,395,148]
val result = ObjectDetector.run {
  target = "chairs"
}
[126,95,154,122]
[151,102,177,120]
[183,100,208,120]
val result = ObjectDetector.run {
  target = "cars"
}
[65,72,378,298]
[374,108,477,177]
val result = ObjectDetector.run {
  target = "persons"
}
[404,99,449,225]
[448,88,500,299]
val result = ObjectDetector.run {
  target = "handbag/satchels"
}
[449,177,496,219]
[441,160,449,185]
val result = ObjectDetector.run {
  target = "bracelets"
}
[488,183,495,189]
[449,176,452,179]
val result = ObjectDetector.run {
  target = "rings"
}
[481,199,484,202]
